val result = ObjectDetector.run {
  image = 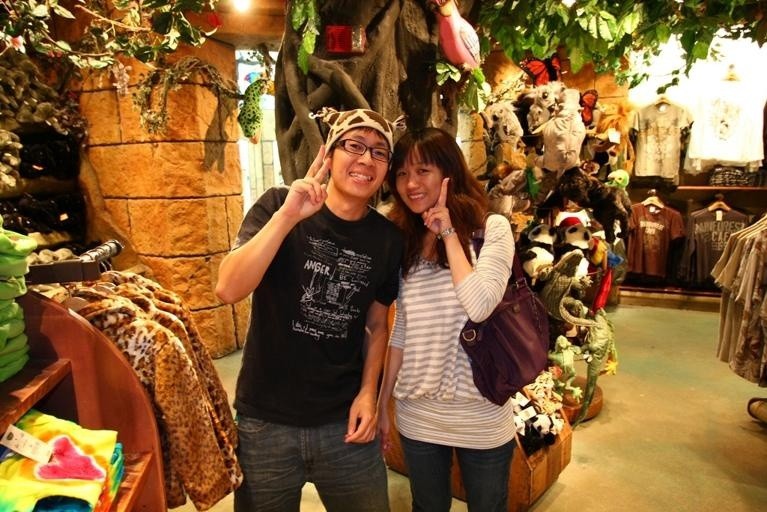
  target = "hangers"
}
[642,189,664,210]
[708,194,730,214]
[25,237,123,283]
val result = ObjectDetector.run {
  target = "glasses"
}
[335,139,390,163]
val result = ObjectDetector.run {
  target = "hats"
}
[308,107,409,159]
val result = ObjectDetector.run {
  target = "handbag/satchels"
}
[460,212,551,402]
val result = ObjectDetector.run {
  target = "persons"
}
[213,108,407,512]
[377,130,552,512]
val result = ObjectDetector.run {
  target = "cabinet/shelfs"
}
[0,290,170,510]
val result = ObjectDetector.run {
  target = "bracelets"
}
[437,227,455,240]
